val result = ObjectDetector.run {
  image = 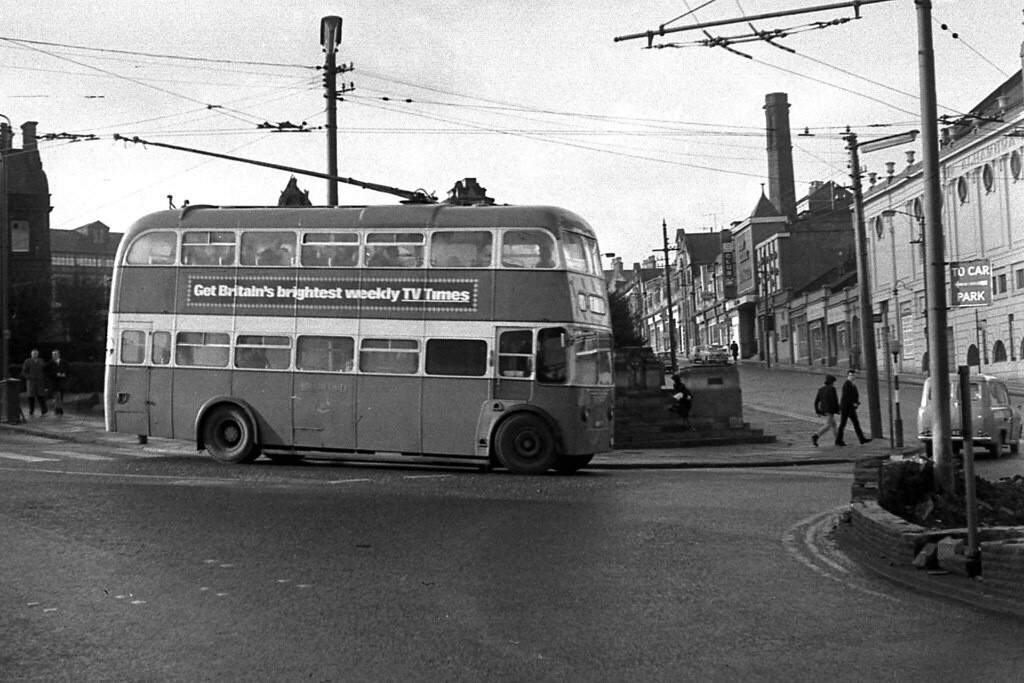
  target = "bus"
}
[104,205,616,476]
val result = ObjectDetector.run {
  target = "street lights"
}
[882,209,930,377]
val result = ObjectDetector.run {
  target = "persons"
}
[24,349,48,415]
[48,349,68,414]
[726,344,730,357]
[730,341,739,361]
[811,375,840,447]
[536,248,555,268]
[672,376,692,424]
[836,370,872,447]
[370,246,404,266]
[260,239,285,264]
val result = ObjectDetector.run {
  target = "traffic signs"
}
[949,258,993,308]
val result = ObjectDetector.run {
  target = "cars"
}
[653,352,679,373]
[687,344,729,365]
[917,373,1023,459]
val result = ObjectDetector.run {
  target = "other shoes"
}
[29,411,33,415]
[812,435,818,447]
[54,407,63,415]
[860,439,873,444]
[41,409,48,415]
[835,440,847,446]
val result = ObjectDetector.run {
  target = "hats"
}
[824,375,837,385]
